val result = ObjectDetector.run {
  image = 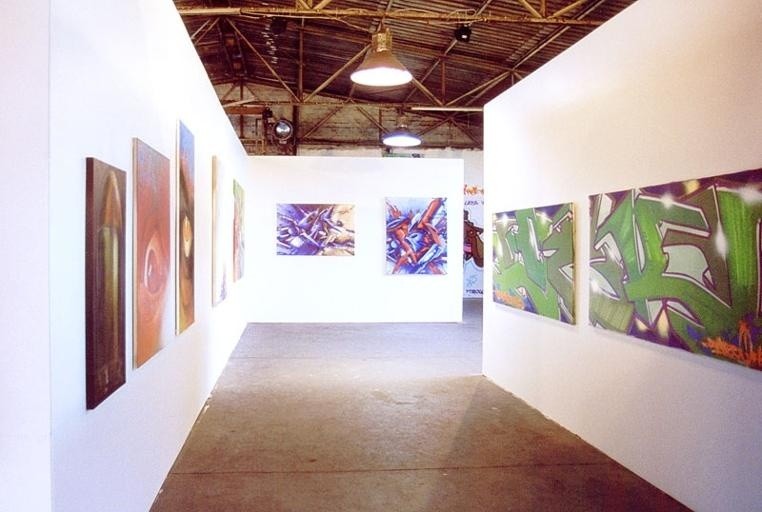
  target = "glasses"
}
[350,22,414,88]
[379,109,423,148]
